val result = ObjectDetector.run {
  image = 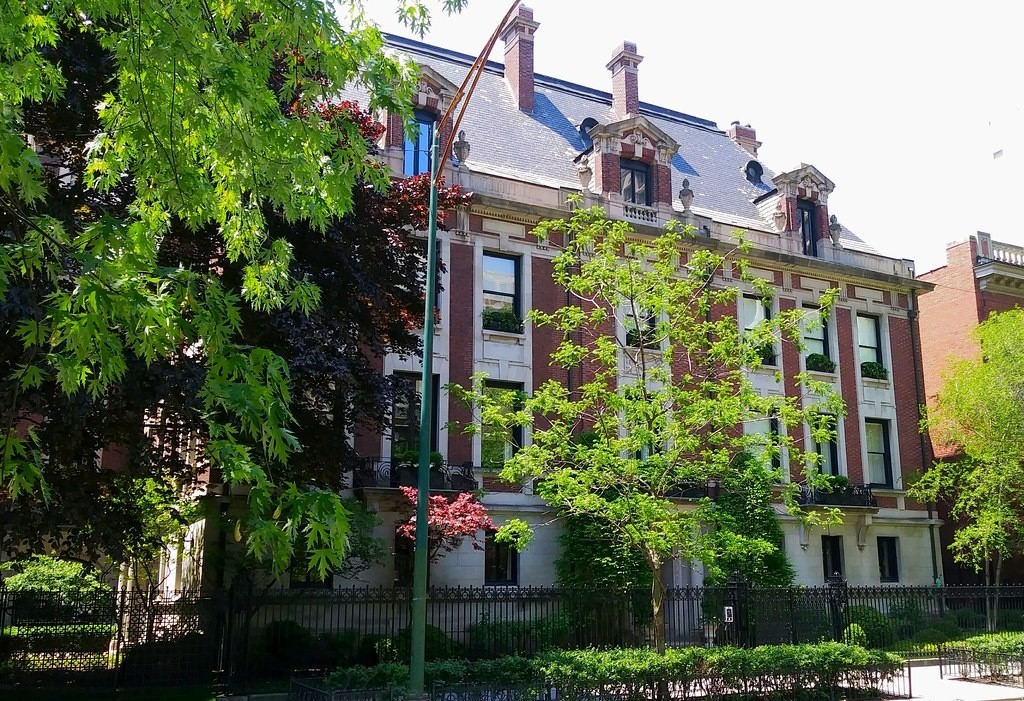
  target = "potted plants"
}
[815,471,856,502]
